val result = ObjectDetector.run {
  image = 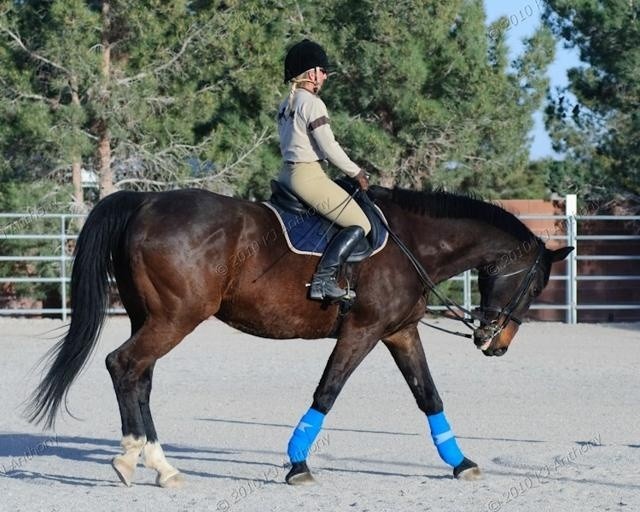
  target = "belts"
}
[283,159,324,165]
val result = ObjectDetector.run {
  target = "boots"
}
[310,225,366,301]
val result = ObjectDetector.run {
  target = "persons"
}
[276,38,372,302]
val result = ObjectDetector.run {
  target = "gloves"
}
[354,168,373,192]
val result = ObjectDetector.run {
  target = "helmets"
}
[282,38,328,85]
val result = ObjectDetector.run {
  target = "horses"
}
[23,186,576,491]
